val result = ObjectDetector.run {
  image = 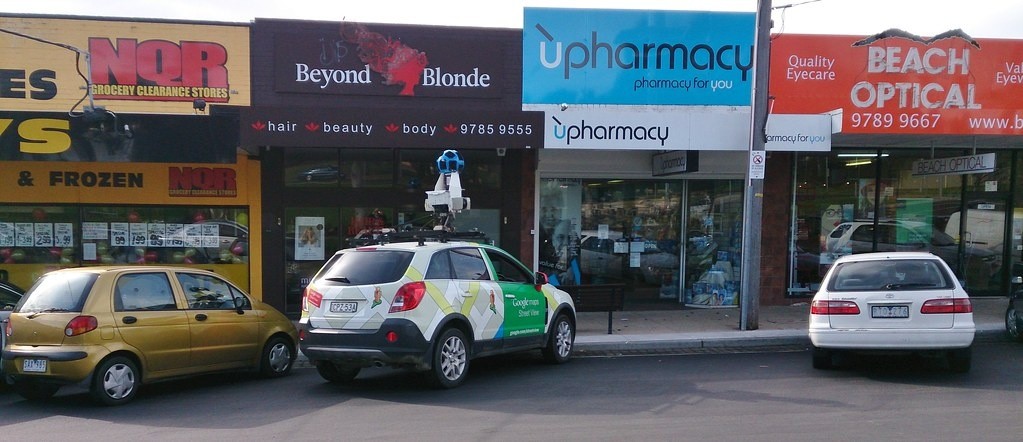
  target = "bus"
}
[575,229,680,284]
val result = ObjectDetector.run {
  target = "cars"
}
[1004,276,1023,341]
[807,251,976,369]
[0,282,24,372]
[2,263,299,407]
[826,218,999,290]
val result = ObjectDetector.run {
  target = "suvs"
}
[296,148,578,389]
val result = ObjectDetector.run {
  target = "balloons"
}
[0,206,247,264]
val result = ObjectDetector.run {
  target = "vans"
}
[944,207,1023,291]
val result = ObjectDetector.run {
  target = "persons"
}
[858,178,887,218]
[712,293,725,306]
[539,179,565,248]
[540,219,579,285]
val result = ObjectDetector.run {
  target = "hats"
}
[860,179,890,196]
